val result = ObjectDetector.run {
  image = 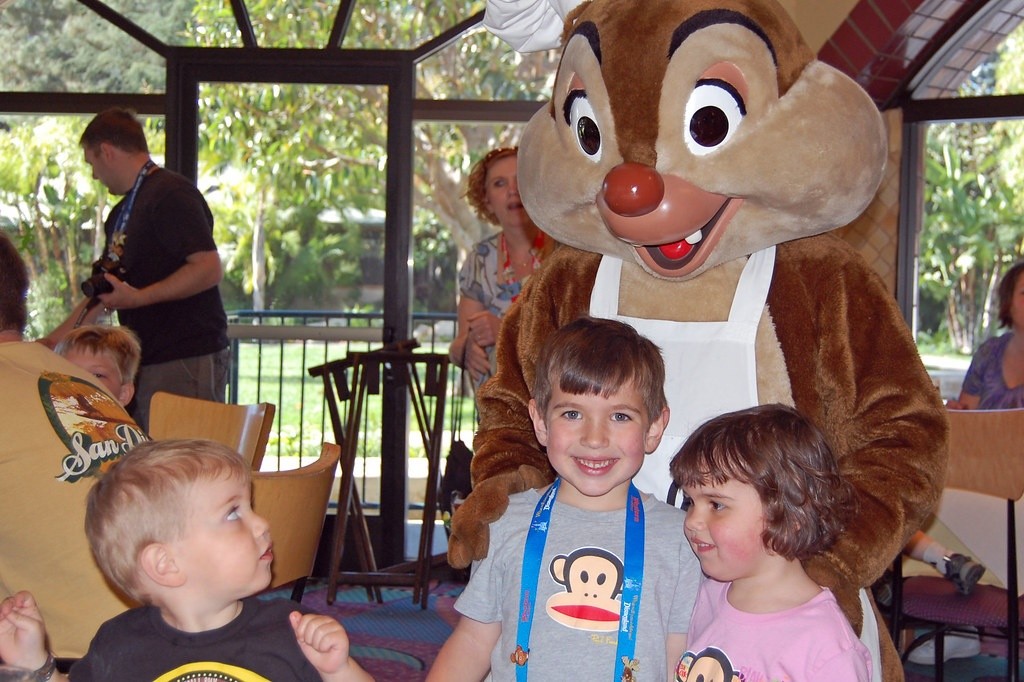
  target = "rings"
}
[478,334,481,340]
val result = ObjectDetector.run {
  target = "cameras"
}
[81,257,134,298]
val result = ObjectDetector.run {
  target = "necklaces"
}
[510,256,531,266]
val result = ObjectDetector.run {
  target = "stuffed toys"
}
[447,0,950,682]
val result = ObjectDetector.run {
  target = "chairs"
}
[143,391,276,470]
[874,408,1024,681]
[250,442,341,601]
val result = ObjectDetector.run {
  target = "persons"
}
[669,403,874,682]
[35,106,231,433]
[0,228,152,674]
[945,262,1024,410]
[425,315,709,682]
[0,437,376,682]
[448,146,561,396]
[901,529,986,596]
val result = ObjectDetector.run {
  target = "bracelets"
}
[35,652,56,680]
